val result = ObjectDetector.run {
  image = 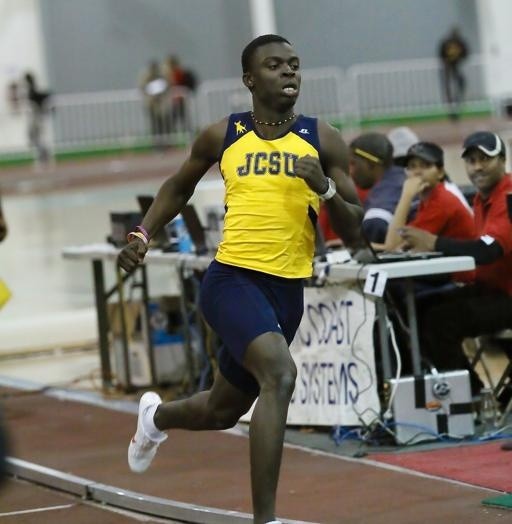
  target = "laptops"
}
[180,203,210,255]
[336,216,444,264]
[137,194,178,248]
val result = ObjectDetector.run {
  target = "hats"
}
[392,142,444,165]
[461,131,506,158]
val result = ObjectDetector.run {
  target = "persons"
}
[138,57,171,150]
[5,80,22,109]
[24,71,59,161]
[437,26,471,121]
[161,53,197,147]
[311,123,512,421]
[116,34,366,523]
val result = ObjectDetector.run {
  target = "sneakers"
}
[127,391,168,473]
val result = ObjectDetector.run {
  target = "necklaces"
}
[250,111,296,126]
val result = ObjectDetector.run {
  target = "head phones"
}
[422,357,450,399]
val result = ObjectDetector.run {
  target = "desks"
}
[61,244,477,396]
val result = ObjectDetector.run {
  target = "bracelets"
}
[135,224,150,239]
[126,231,148,245]
